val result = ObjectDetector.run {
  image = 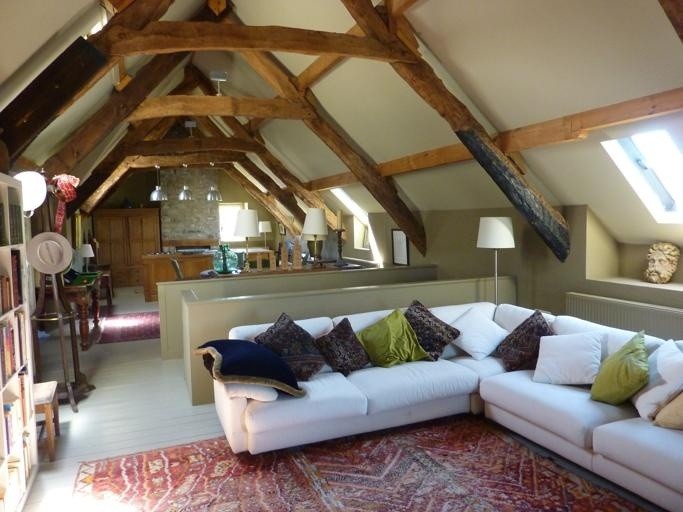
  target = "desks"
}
[29,268,104,351]
[197,260,362,280]
[80,261,113,314]
[137,245,271,304]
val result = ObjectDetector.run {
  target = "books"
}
[1,202,32,512]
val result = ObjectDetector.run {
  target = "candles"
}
[334,207,343,231]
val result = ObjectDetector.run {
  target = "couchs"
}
[187,295,497,460]
[474,299,683,511]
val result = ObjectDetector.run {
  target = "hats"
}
[27,232,72,274]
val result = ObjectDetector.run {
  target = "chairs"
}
[167,251,186,281]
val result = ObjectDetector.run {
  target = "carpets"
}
[70,412,658,511]
[93,311,161,346]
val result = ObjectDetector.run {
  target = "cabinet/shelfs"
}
[0,171,43,512]
[91,208,127,288]
[123,205,162,289]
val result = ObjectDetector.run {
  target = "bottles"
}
[255,239,307,271]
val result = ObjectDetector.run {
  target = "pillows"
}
[188,337,306,400]
[251,312,328,385]
[446,304,683,431]
[355,306,433,368]
[400,297,461,364]
[311,314,369,381]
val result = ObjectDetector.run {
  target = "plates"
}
[177,248,209,255]
[199,268,241,279]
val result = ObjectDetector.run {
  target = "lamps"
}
[184,120,198,138]
[76,242,95,273]
[233,209,260,274]
[474,213,517,306]
[301,206,329,261]
[146,154,223,204]
[257,220,272,250]
[208,70,228,98]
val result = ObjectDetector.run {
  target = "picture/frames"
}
[277,220,286,237]
[388,227,409,267]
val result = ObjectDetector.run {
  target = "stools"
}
[30,378,62,463]
